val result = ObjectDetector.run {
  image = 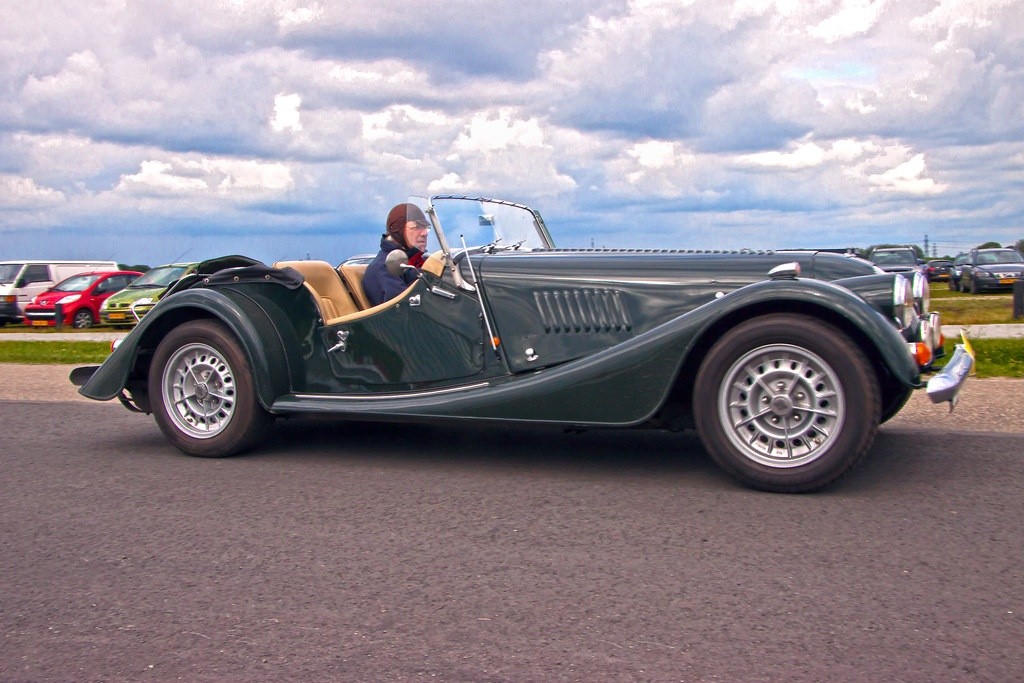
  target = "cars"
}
[861,236,1023,294]
[99,264,197,330]
[21,271,142,337]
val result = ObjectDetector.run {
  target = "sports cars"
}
[71,190,975,494]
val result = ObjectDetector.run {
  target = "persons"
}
[358,203,440,320]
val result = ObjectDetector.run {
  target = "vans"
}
[1,256,120,331]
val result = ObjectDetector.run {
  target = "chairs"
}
[274,258,379,323]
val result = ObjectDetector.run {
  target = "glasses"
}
[405,223,431,233]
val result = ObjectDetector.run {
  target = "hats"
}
[386,203,431,249]
